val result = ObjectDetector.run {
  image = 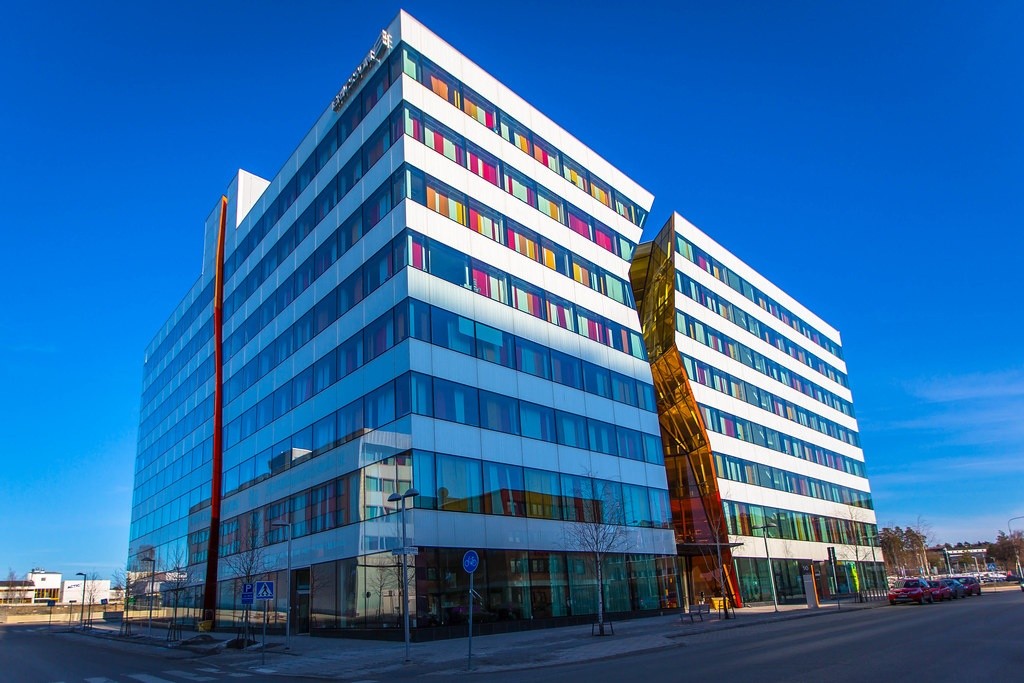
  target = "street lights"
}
[387,489,420,662]
[76,573,87,628]
[752,523,781,615]
[141,557,156,637]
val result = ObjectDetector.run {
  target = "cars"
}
[941,579,965,599]
[953,576,982,596]
[888,579,934,605]
[926,580,953,601]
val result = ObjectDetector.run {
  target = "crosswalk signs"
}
[256,581,274,600]
